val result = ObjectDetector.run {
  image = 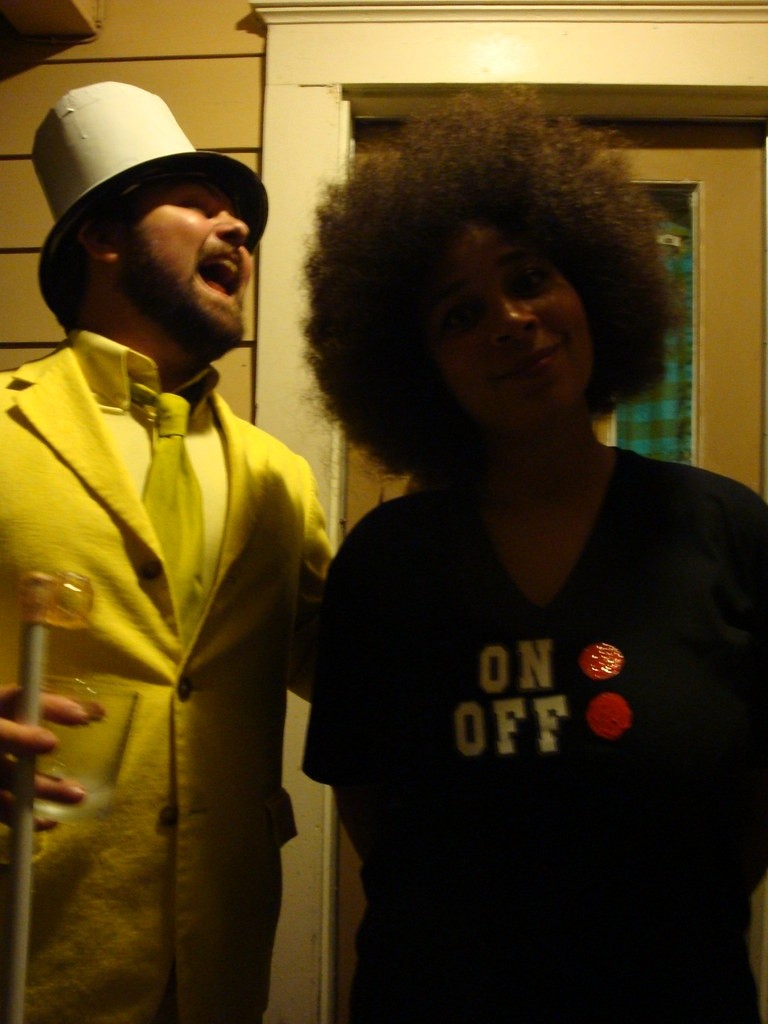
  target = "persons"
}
[0,82,332,1024]
[300,85,768,1024]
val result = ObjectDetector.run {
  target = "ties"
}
[127,380,213,650]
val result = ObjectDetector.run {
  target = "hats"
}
[31,80,269,336]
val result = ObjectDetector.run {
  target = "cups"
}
[33,676,139,820]
